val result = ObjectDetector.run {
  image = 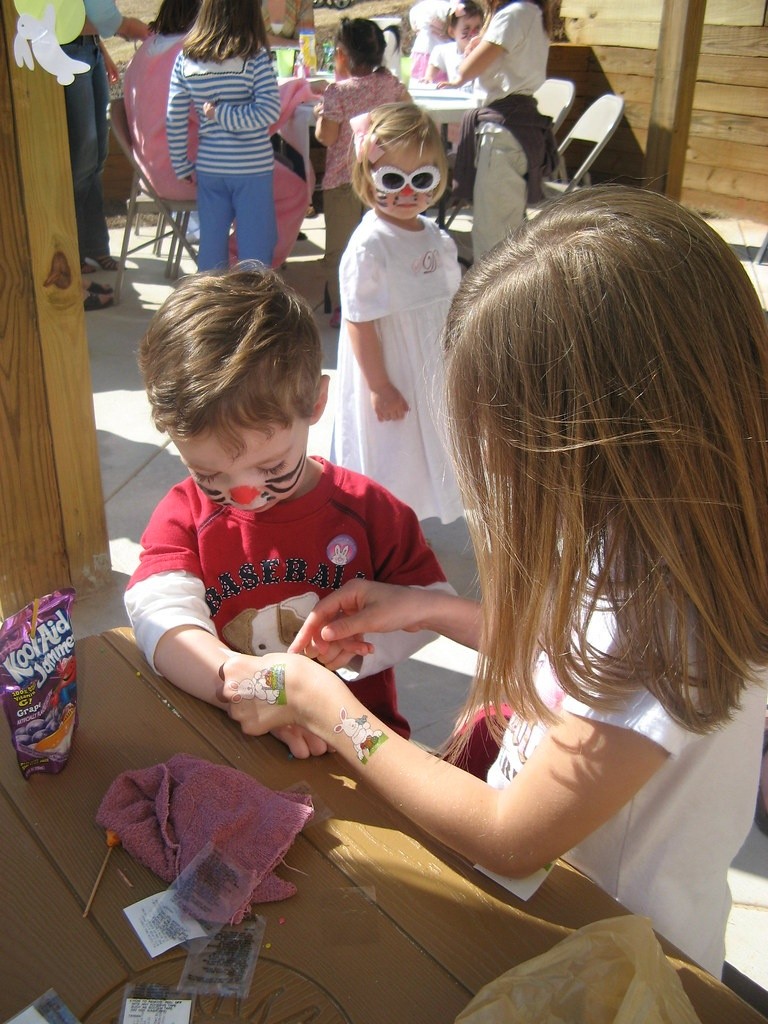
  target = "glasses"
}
[365,166,442,193]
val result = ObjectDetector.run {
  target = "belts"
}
[72,35,100,45]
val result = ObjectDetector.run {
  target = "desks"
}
[0,627,768,1024]
[274,71,477,314]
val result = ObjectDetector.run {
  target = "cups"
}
[276,49,295,77]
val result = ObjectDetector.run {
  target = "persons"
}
[328,103,466,525]
[124,270,456,758]
[314,17,413,328]
[61,0,156,271]
[82,278,114,310]
[125,0,328,270]
[409,0,563,263]
[220,186,768,981]
[167,0,282,275]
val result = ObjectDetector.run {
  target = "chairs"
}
[105,96,204,306]
[435,78,625,231]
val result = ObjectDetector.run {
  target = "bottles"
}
[299,29,316,78]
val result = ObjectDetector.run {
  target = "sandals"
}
[81,261,95,273]
[94,255,126,273]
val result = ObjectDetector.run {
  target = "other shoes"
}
[329,307,341,327]
[84,291,113,310]
[87,281,114,293]
